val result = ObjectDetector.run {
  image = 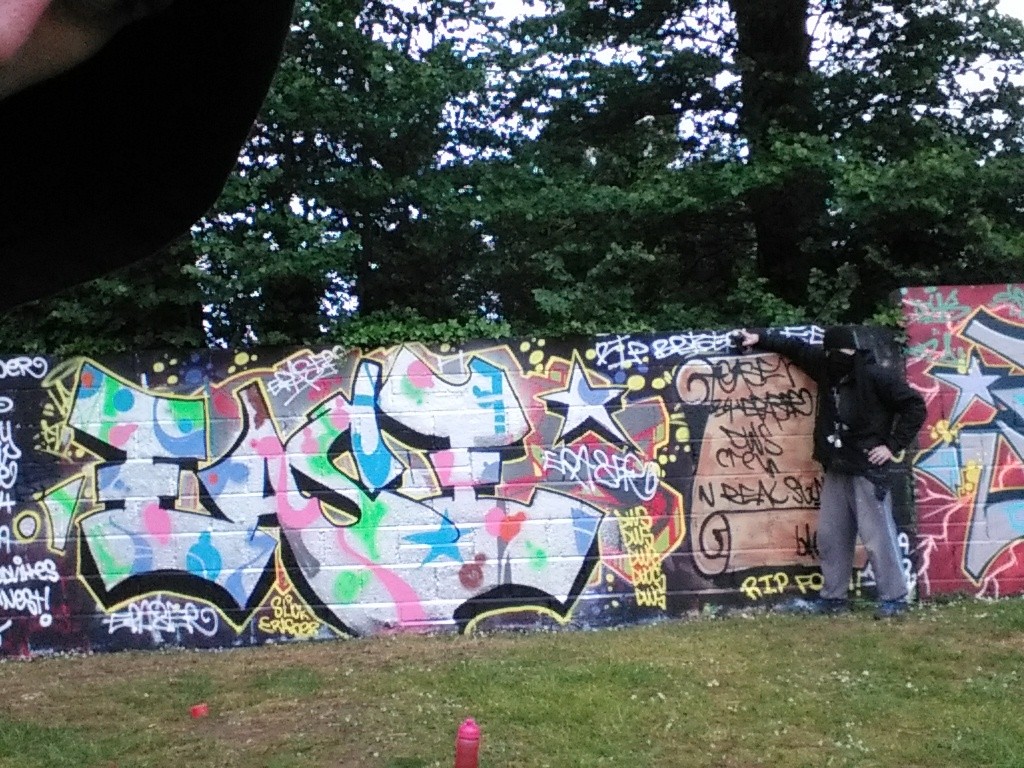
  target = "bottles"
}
[454,719,481,768]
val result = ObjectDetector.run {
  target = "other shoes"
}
[875,601,910,619]
[812,598,849,616]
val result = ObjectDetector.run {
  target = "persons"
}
[729,326,928,619]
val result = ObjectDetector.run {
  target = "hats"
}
[822,326,857,349]
